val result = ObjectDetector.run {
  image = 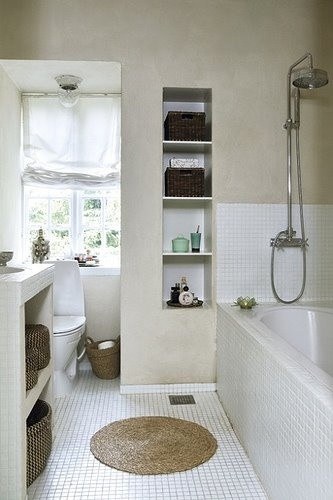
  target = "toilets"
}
[42,259,86,393]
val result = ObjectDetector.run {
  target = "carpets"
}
[88,415,219,477]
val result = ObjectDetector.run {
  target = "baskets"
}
[165,167,205,197]
[26,399,52,488]
[26,349,38,390]
[85,335,120,380]
[164,111,206,141]
[25,324,50,369]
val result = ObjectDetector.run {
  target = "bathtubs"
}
[261,305,332,380]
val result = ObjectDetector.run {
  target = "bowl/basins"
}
[0,251,13,266]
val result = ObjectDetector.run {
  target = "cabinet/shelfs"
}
[161,86,214,311]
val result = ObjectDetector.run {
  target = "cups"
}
[191,233,201,253]
